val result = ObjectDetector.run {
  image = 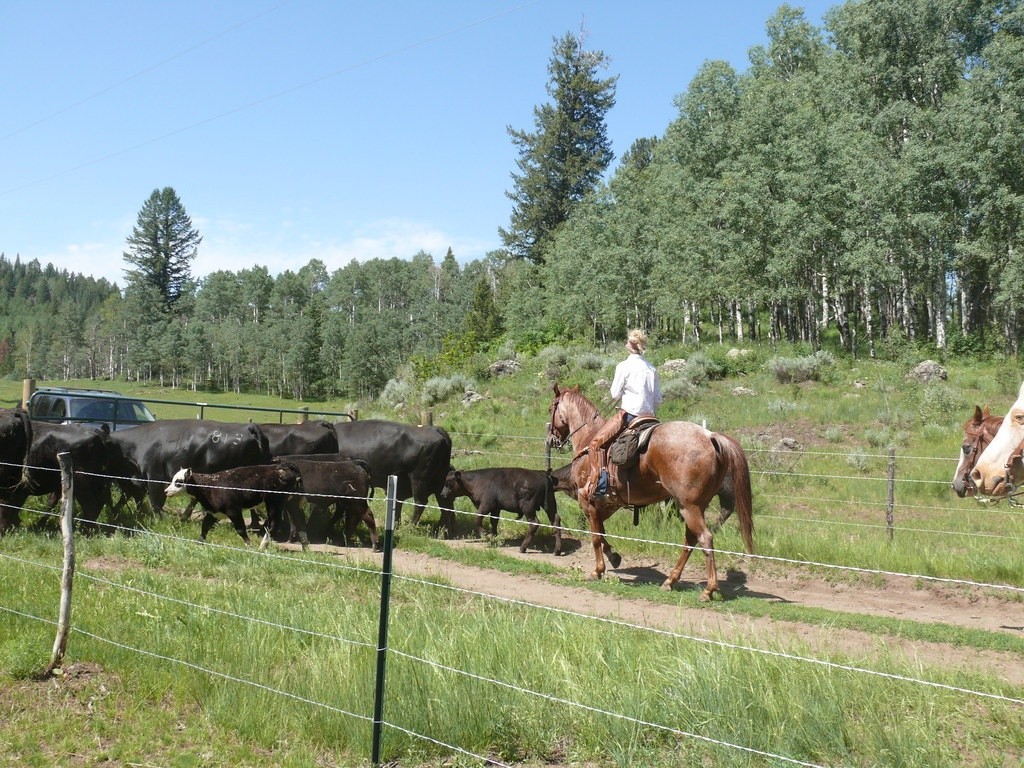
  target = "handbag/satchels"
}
[611,429,638,468]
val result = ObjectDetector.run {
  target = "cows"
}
[0,407,575,556]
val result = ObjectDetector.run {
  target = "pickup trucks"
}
[24,386,157,434]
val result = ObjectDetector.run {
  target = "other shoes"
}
[579,487,607,507]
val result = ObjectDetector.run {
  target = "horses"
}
[950,381,1024,498]
[546,383,757,603]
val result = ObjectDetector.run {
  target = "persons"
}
[578,329,662,502]
